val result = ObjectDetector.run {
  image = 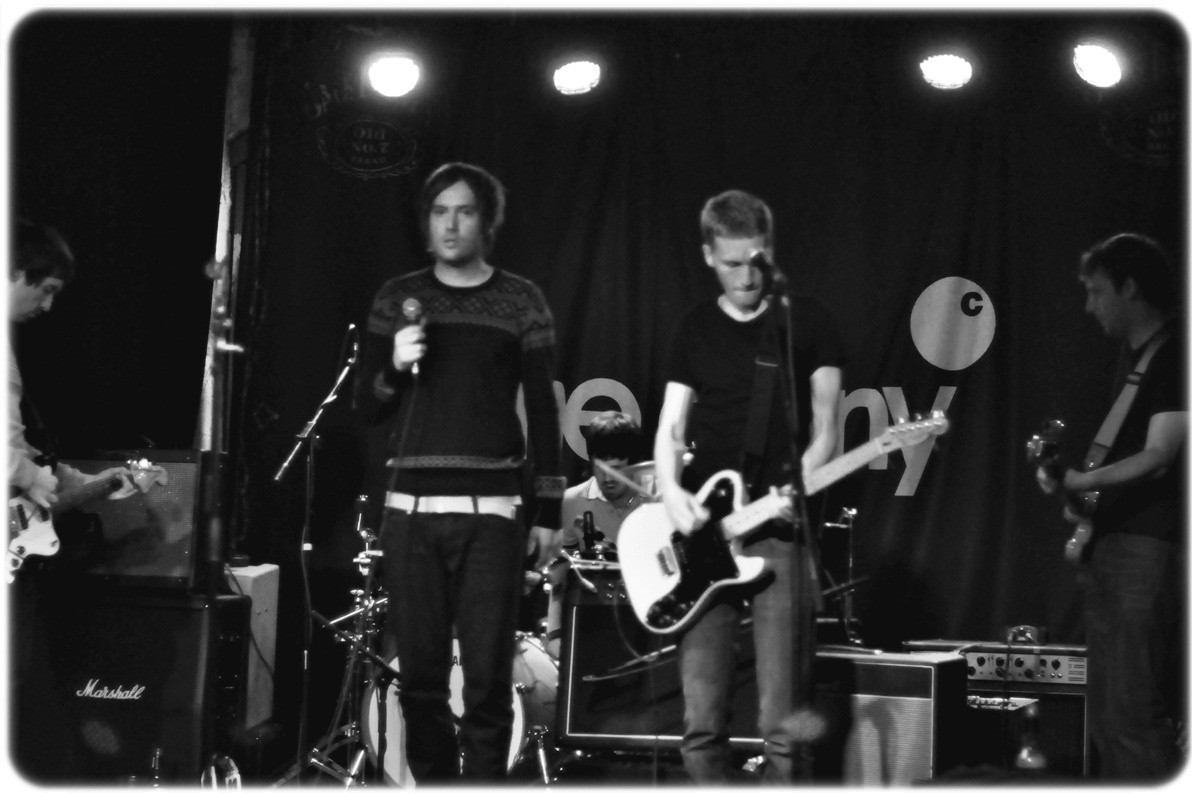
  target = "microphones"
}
[573,511,617,552]
[403,298,421,377]
[1016,705,1043,769]
[608,644,677,675]
[744,247,788,287]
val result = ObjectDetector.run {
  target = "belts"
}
[384,489,522,519]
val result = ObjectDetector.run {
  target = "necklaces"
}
[626,496,634,506]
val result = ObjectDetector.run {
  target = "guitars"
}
[1023,431,1104,572]
[2,449,172,588]
[614,409,950,635]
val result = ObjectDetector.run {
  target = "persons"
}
[9,224,138,509]
[562,410,659,551]
[358,162,563,788]
[655,189,847,783]
[1061,233,1190,781]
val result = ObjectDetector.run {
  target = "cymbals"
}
[617,447,695,476]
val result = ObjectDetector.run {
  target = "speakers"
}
[16,447,234,596]
[10,587,253,789]
[903,635,1093,779]
[555,573,770,749]
[800,649,967,790]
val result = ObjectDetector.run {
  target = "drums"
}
[359,639,529,784]
[543,548,630,662]
